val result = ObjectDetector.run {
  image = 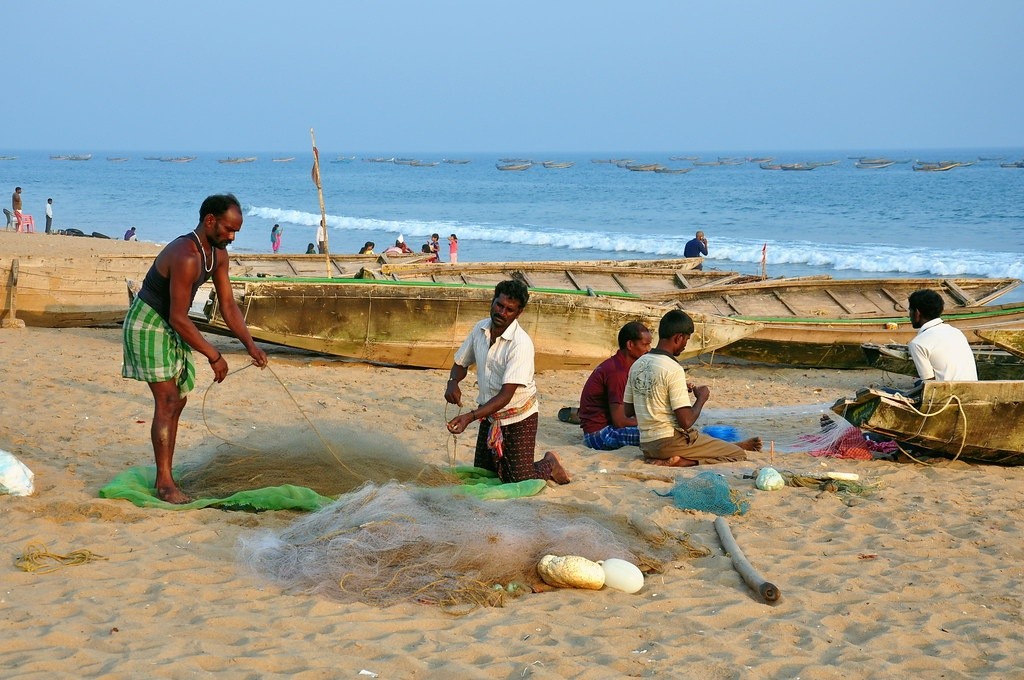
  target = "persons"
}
[11,186,23,232]
[356,240,413,254]
[624,310,750,467]
[882,289,979,400]
[271,223,284,254]
[683,231,708,271]
[443,280,573,486]
[448,234,459,263]
[317,220,329,254]
[421,244,435,263]
[428,233,440,262]
[45,198,53,234]
[124,226,138,241]
[579,322,763,453]
[119,193,272,506]
[305,243,317,254]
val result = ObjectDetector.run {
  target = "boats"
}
[1,228,1023,382]
[827,378,1024,472]
[0,148,1023,178]
[204,277,762,373]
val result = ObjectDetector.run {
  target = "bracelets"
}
[448,377,458,382]
[208,350,222,364]
[471,410,478,420]
